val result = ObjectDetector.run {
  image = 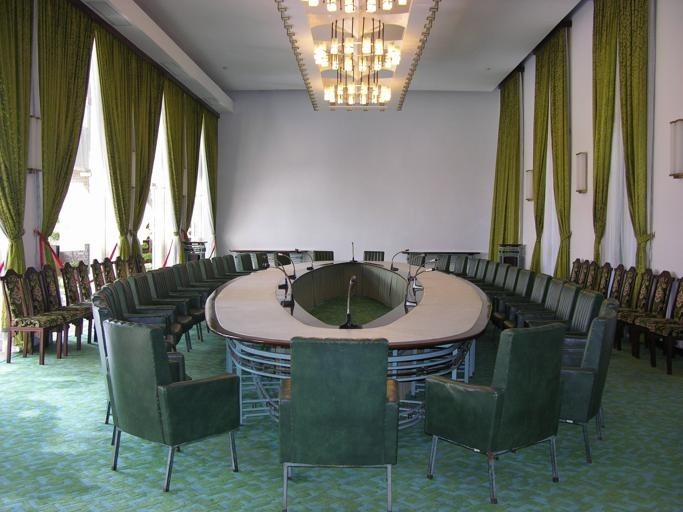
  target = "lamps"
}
[669,120,683,179]
[525,170,534,200]
[272,1,441,112]
[575,152,588,193]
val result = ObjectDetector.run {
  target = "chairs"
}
[406,252,682,504]
[312,251,334,261]
[279,334,398,510]
[363,251,384,261]
[0,252,292,492]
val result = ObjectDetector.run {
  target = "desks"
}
[206,259,493,432]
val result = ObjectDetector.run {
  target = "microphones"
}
[350,242,358,262]
[278,253,296,279]
[404,268,436,314]
[413,258,438,296]
[391,251,409,271]
[264,256,288,298]
[407,253,427,281]
[338,275,363,329]
[297,251,314,270]
[262,263,295,315]
